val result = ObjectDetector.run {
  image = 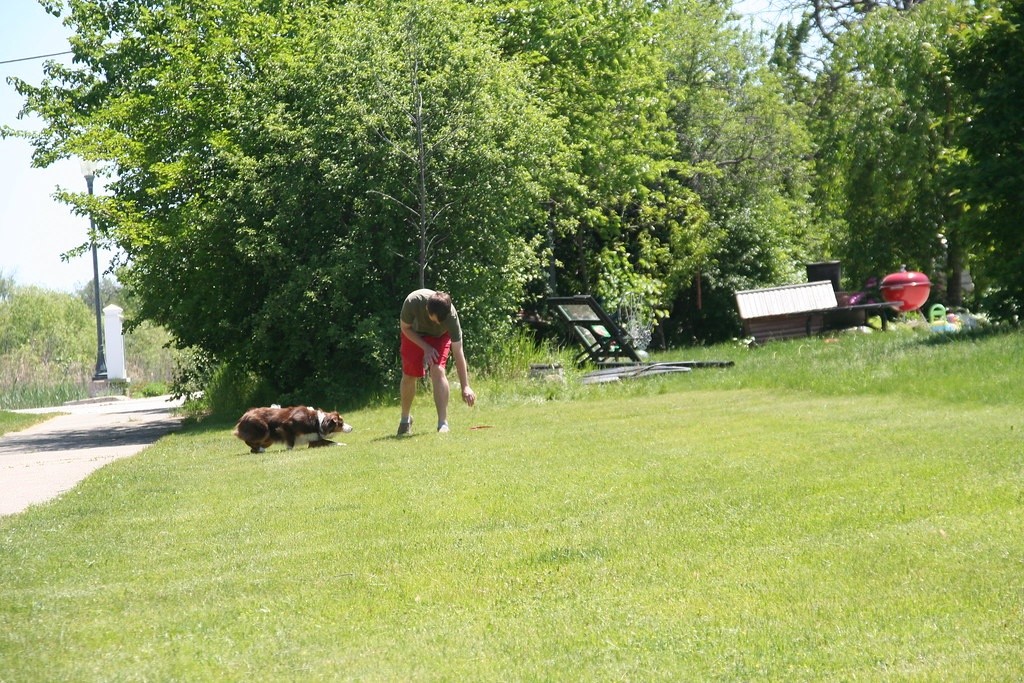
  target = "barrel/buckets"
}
[806,259,841,291]
[529,362,566,387]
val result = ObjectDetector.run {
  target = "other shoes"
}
[437,421,450,434]
[397,416,414,437]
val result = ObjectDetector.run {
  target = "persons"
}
[397,289,476,436]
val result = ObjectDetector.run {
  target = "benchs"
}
[803,301,904,339]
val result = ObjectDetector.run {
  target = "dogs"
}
[234,407,349,452]
